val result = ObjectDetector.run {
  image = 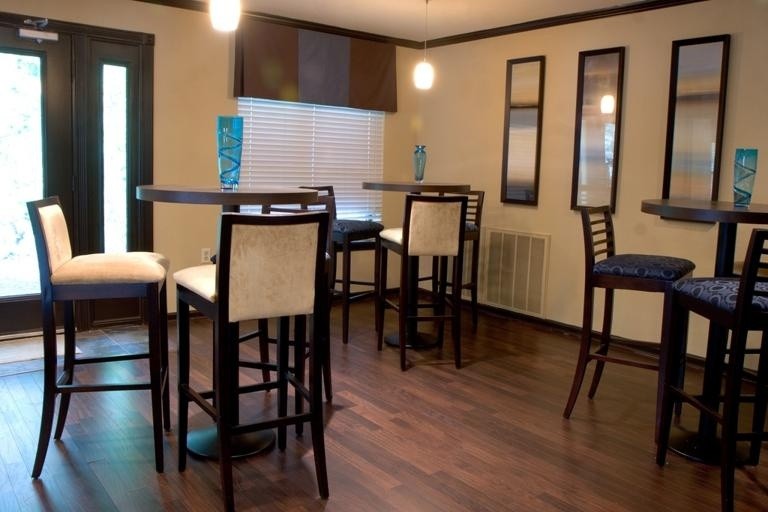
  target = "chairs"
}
[297,185,384,343]
[417,189,486,337]
[173,211,332,510]
[656,227,767,511]
[376,193,469,371]
[562,205,696,444]
[26,194,170,480]
[172,194,337,454]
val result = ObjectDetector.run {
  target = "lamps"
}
[411,0,436,92]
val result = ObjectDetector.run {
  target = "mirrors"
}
[662,32,732,223]
[499,53,547,208]
[568,45,626,214]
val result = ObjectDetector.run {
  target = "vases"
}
[412,141,428,182]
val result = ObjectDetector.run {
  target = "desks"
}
[136,184,319,463]
[640,198,768,466]
[362,182,471,351]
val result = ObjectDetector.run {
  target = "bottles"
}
[413,144,426,184]
